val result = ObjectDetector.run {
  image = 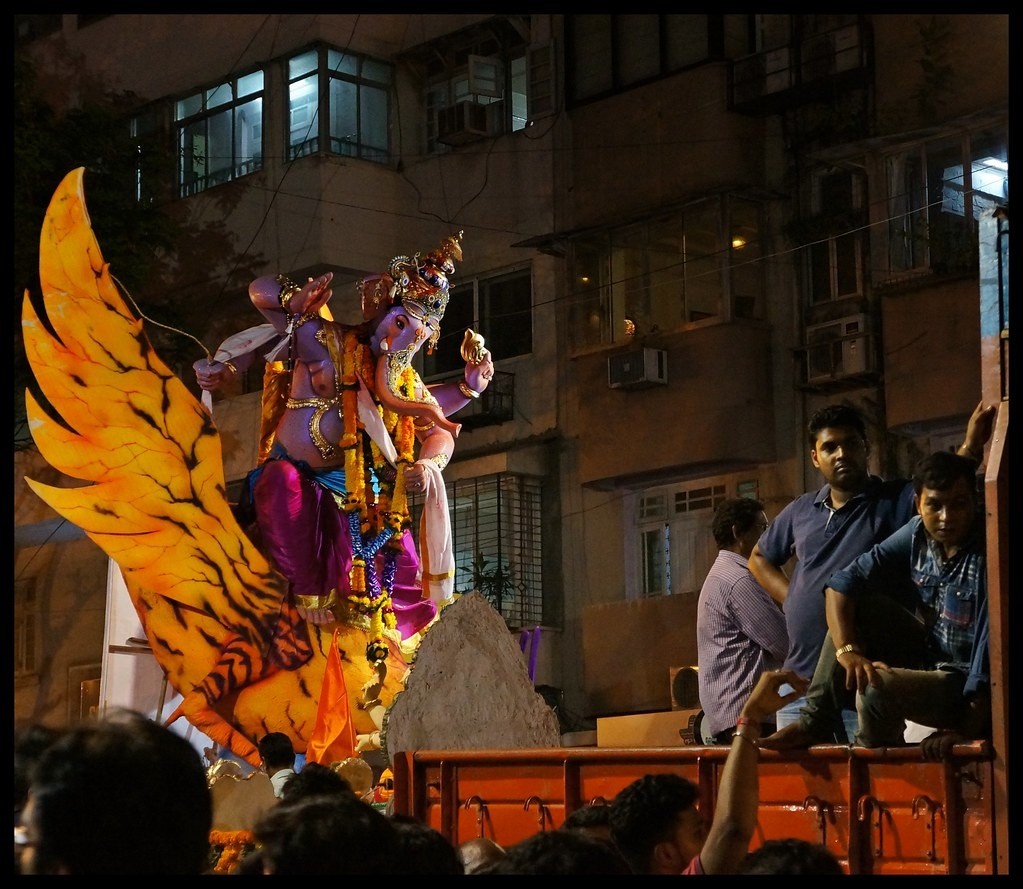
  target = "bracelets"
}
[835,644,861,662]
[737,718,759,731]
[731,732,760,753]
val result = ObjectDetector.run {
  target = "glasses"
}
[748,522,770,534]
[12,826,39,855]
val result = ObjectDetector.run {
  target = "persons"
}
[13,669,848,876]
[746,398,996,743]
[696,499,791,743]
[757,449,989,746]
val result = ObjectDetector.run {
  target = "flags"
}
[306,627,357,764]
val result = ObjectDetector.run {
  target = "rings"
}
[774,669,781,672]
